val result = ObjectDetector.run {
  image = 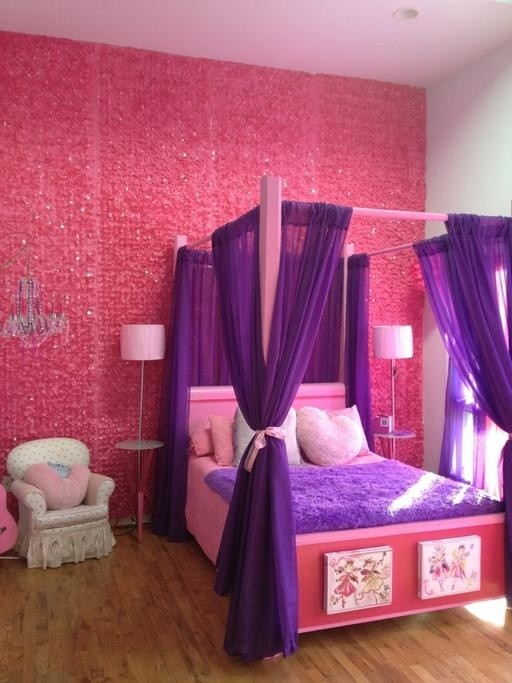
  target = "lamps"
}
[373,324,416,433]
[2,243,69,351]
[120,322,166,445]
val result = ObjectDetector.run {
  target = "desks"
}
[373,427,418,459]
[114,437,170,540]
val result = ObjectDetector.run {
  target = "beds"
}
[172,172,512,659]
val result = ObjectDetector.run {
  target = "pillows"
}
[185,403,373,475]
[24,461,91,511]
[46,460,70,479]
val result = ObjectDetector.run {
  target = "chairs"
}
[5,435,119,570]
